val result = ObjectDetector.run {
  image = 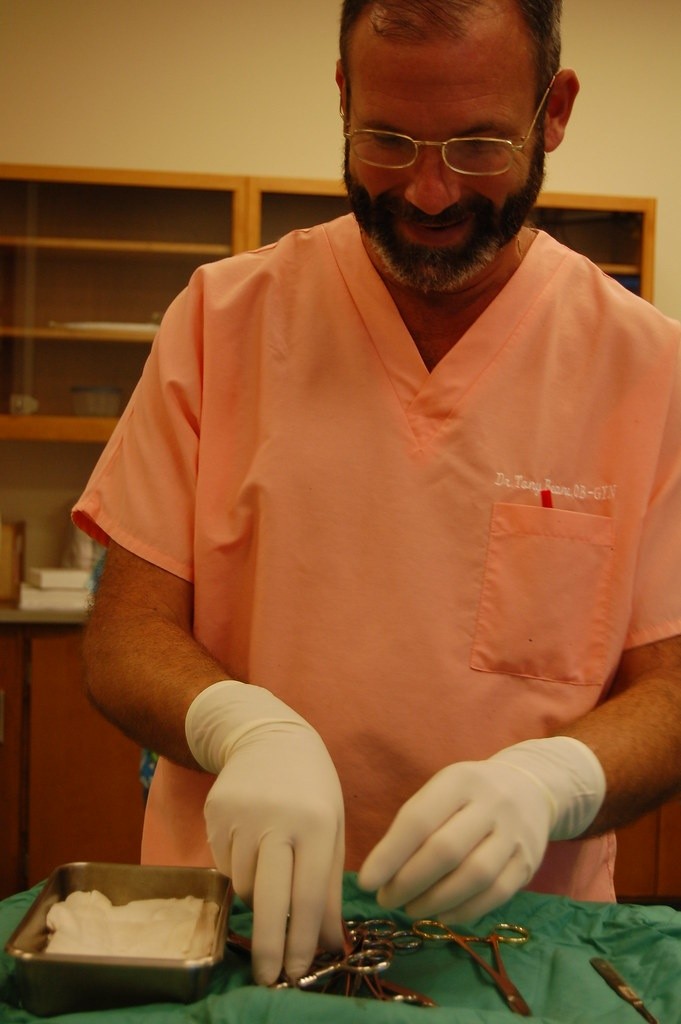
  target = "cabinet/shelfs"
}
[0,161,681,908]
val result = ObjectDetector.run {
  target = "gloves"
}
[184,681,345,986]
[357,736,605,927]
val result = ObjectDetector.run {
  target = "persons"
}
[71,1,680,987]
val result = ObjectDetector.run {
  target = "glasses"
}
[339,68,563,176]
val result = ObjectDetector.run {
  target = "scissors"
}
[412,918,531,1017]
[266,918,440,1009]
